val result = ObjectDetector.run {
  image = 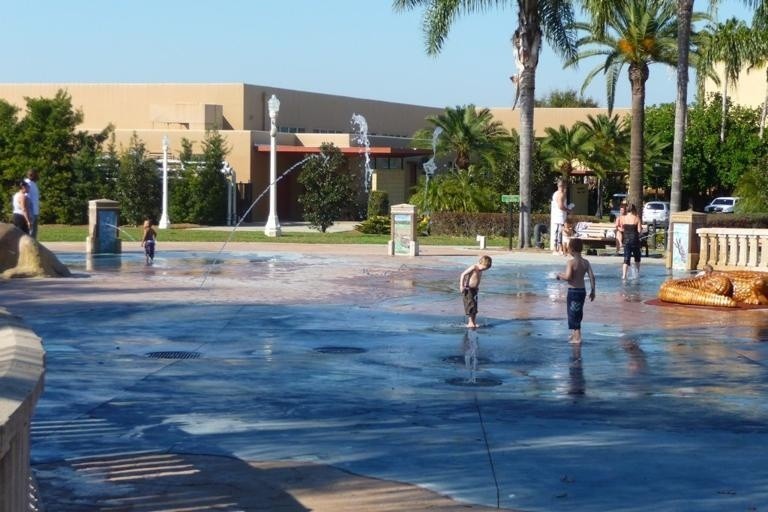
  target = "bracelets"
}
[27,221,31,224]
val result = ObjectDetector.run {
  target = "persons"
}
[615,206,628,255]
[555,238,595,344]
[560,219,573,257]
[23,168,39,239]
[459,255,491,327]
[12,180,32,235]
[619,204,641,281]
[568,341,588,395]
[140,219,157,263]
[550,180,570,254]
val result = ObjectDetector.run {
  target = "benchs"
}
[571,221,649,257]
